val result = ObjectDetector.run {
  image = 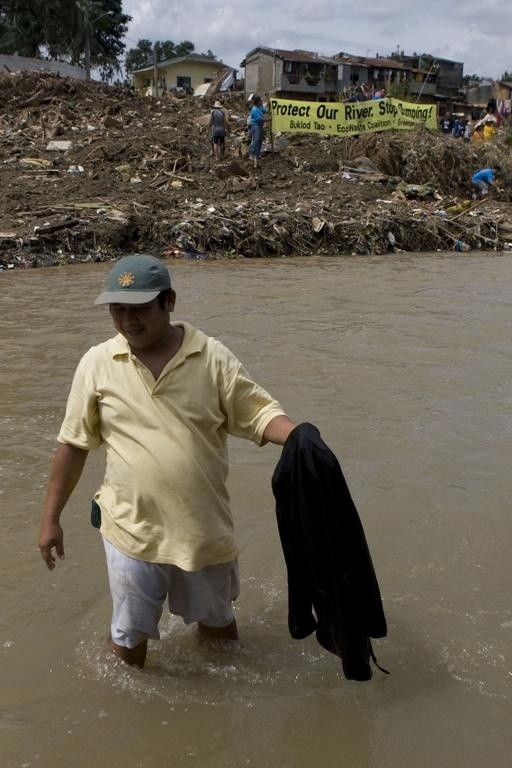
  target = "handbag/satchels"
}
[90,496,102,527]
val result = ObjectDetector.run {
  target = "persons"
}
[205,101,231,161]
[470,165,505,199]
[39,252,303,674]
[246,95,273,160]
[351,87,368,102]
[435,108,498,143]
[372,88,385,101]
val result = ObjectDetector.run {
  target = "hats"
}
[213,100,223,109]
[94,254,171,305]
